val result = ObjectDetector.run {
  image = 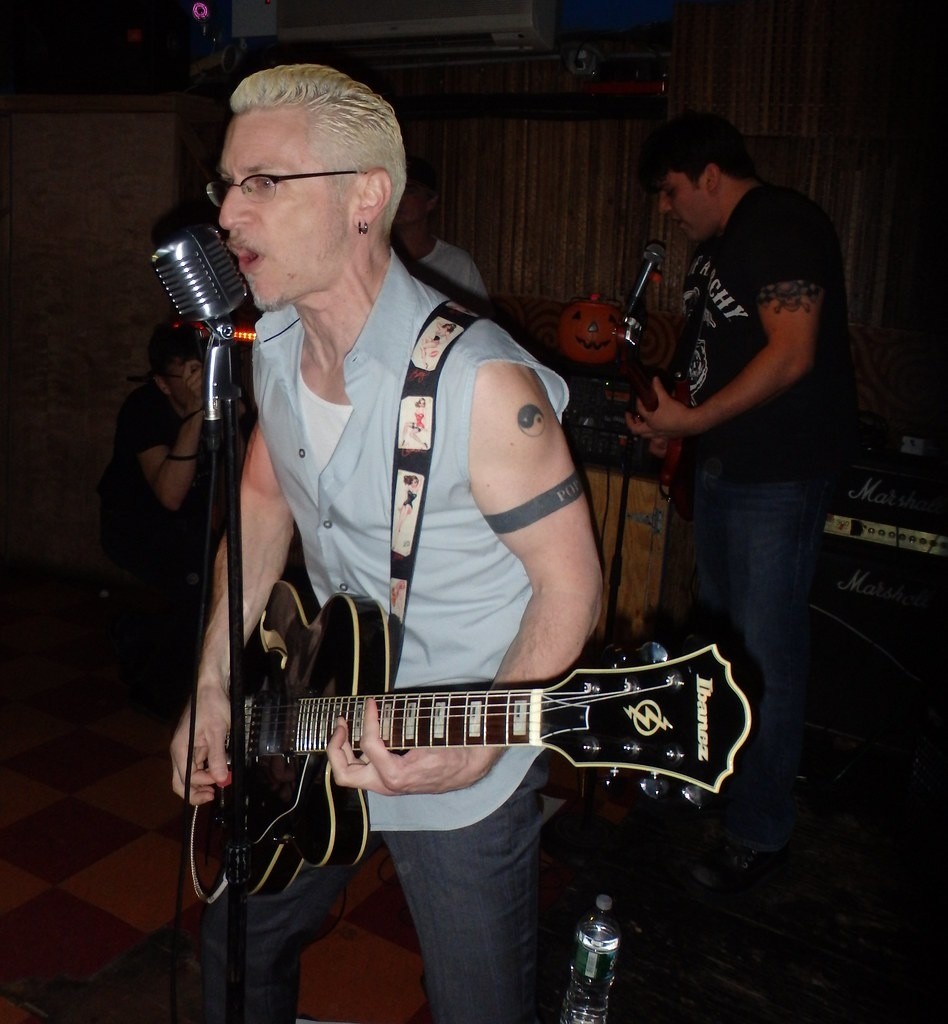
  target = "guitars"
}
[186,576,758,909]
[613,317,700,525]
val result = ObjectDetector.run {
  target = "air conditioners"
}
[277,0,557,52]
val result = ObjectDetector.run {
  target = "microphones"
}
[151,225,246,451]
[625,244,666,319]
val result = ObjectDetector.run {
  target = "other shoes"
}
[689,842,795,895]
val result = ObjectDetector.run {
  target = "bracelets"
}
[166,453,199,461]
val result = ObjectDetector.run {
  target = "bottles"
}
[561,895,620,1024]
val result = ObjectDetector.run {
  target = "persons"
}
[98,321,259,724]
[604,111,849,891]
[167,62,607,1024]
[392,154,491,310]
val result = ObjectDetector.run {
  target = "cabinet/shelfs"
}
[576,464,694,665]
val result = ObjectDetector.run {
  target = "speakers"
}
[810,466,948,645]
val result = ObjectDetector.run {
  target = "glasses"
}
[206,170,368,208]
